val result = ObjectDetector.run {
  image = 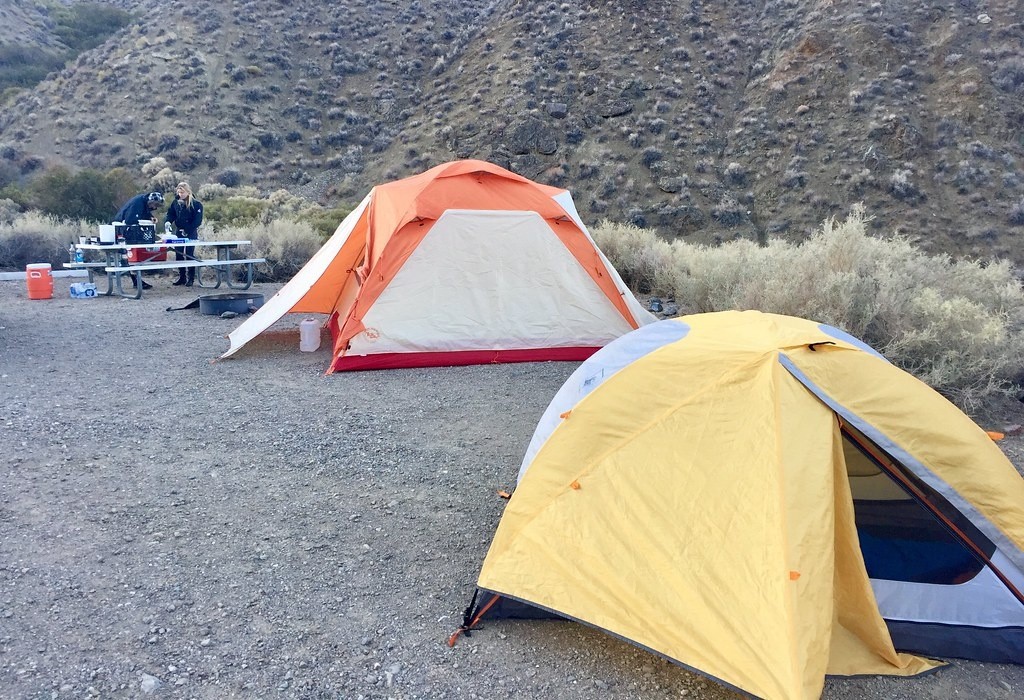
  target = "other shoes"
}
[133,278,153,289]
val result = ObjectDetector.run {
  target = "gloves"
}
[164,221,171,229]
[179,229,188,237]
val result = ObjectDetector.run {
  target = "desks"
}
[62,240,266,299]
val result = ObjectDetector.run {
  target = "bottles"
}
[76,248,83,263]
[68,242,76,263]
[70,282,98,299]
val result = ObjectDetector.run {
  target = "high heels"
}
[173,278,186,286]
[186,279,193,287]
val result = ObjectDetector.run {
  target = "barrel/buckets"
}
[299,317,322,352]
[26,263,53,300]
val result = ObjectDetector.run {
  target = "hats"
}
[147,191,164,206]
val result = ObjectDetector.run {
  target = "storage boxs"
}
[115,225,155,245]
[99,225,115,242]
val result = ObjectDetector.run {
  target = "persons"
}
[112,190,165,289]
[163,182,204,287]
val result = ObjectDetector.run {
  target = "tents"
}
[448,308,1024,700]
[204,159,664,379]
